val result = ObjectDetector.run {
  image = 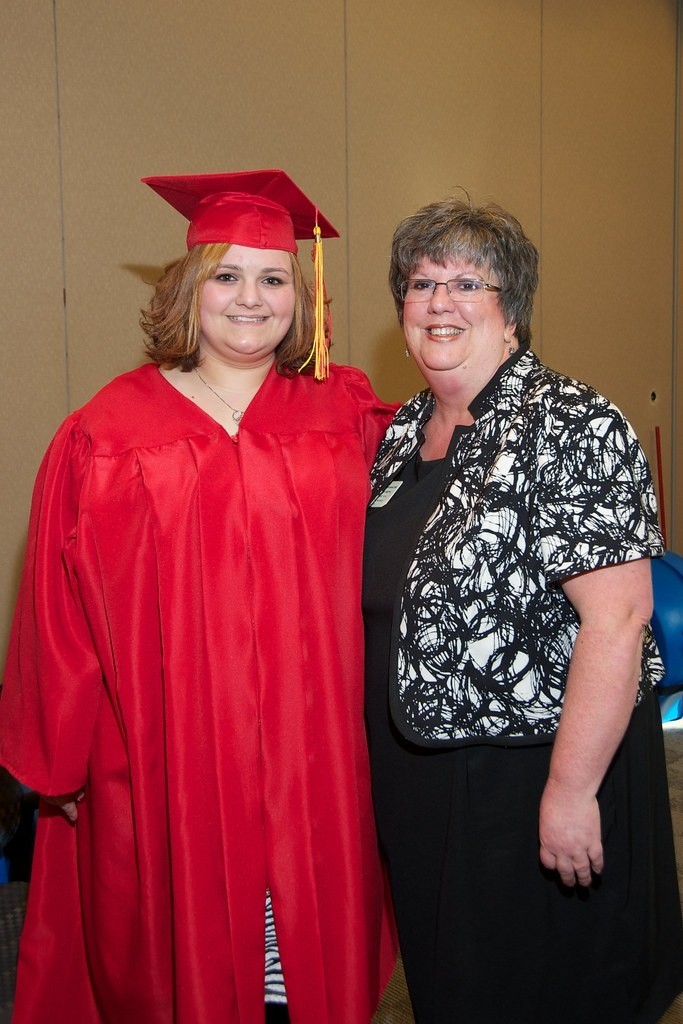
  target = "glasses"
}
[396,276,505,304]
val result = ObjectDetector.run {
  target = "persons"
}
[1,169,406,1024]
[362,196,683,1024]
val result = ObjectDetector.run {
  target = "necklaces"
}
[194,357,261,428]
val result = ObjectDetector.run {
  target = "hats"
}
[141,169,341,382]
